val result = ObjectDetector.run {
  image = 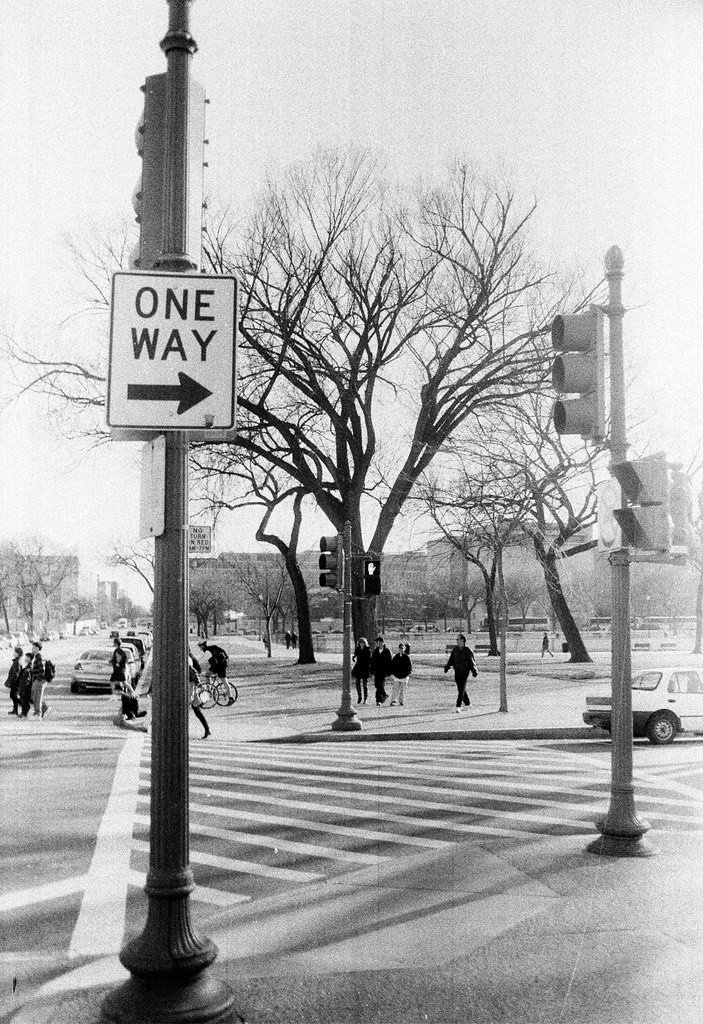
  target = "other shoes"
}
[363,699,367,704]
[202,732,211,739]
[376,700,381,706]
[42,707,48,717]
[400,702,404,707]
[455,707,461,713]
[8,711,17,715]
[390,701,396,706]
[357,698,362,704]
[228,698,235,705]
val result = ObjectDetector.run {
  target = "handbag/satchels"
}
[197,685,211,705]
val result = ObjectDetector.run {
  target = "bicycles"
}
[196,673,238,710]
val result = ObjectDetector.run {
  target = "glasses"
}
[360,642,365,644]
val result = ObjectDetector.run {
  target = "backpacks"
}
[43,657,55,682]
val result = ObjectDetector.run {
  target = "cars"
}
[583,665,703,743]
[45,618,154,694]
[388,626,464,632]
[221,624,261,636]
[311,628,344,634]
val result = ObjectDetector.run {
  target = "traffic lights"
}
[609,452,674,554]
[364,560,383,596]
[549,306,606,439]
[318,534,344,591]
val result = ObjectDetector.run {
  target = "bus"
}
[581,615,643,631]
[376,618,414,631]
[644,616,698,630]
[506,616,551,632]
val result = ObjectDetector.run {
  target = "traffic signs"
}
[105,270,241,442]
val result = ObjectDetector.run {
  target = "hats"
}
[374,637,384,641]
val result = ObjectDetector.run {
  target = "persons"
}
[351,637,412,706]
[285,630,297,649]
[444,634,478,713]
[542,633,553,657]
[4,642,48,717]
[189,641,234,739]
[109,639,147,719]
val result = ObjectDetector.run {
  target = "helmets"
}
[197,640,206,646]
